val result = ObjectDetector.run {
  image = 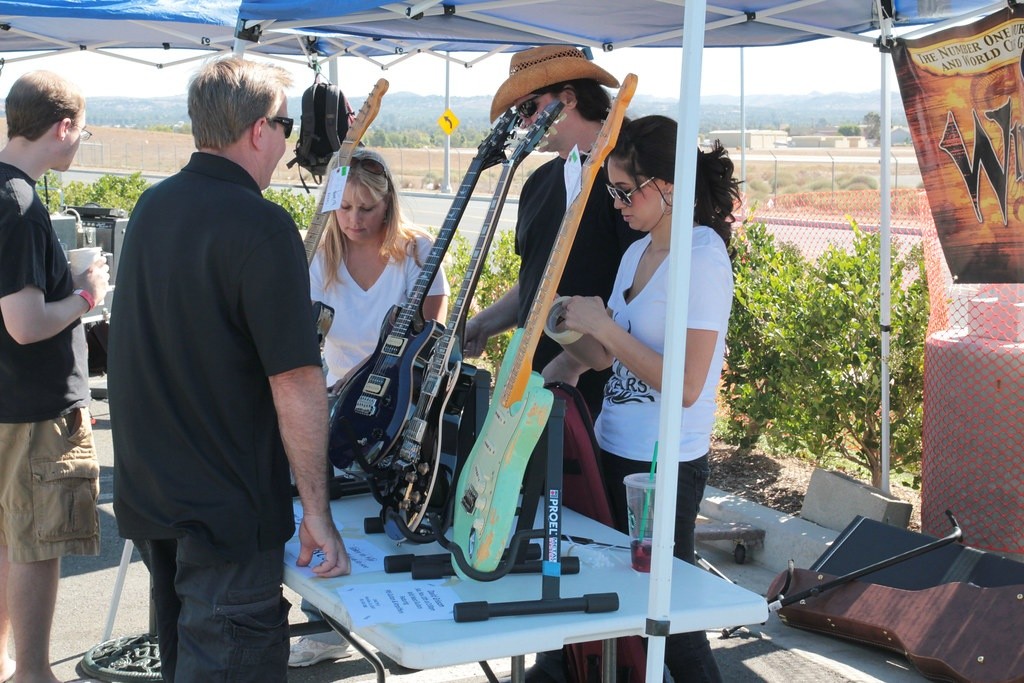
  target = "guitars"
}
[301,78,390,372]
[452,72,640,582]
[326,107,527,477]
[382,97,567,544]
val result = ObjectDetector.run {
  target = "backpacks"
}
[287,74,356,174]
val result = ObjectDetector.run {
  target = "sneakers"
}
[286,636,353,667]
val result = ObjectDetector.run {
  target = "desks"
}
[103,489,767,683]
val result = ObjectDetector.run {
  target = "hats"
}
[489,43,619,124]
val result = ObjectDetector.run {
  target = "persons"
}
[462,46,628,683]
[106,58,351,683]
[565,116,737,683]
[0,72,110,683]
[286,151,449,667]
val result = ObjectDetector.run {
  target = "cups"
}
[623,473,657,573]
[67,247,104,305]
[544,294,614,371]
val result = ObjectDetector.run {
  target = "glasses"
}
[268,116,293,139]
[349,157,390,193]
[59,117,92,141]
[606,177,656,207]
[516,91,550,119]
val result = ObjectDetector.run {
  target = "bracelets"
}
[73,290,95,314]
